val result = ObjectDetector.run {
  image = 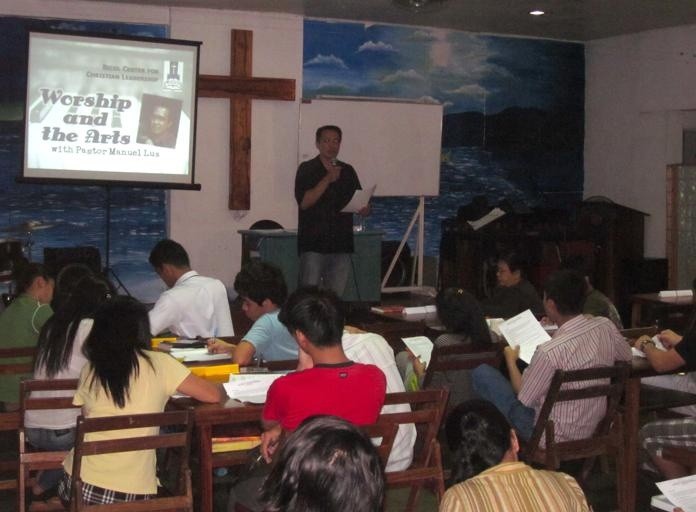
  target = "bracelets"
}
[640,340,656,353]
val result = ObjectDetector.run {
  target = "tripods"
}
[95,187,131,296]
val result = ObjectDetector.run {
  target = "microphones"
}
[331,156,337,166]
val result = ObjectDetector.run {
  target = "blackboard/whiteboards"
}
[298,94,444,197]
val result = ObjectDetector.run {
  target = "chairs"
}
[44,246,131,297]
[247,220,284,230]
[1,293,694,512]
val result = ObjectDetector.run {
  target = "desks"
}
[239,229,384,302]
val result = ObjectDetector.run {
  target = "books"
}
[339,184,376,214]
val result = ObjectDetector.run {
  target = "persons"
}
[1,237,234,512]
[634,277,696,480]
[292,126,372,300]
[204,261,417,512]
[411,241,632,512]
[145,104,179,148]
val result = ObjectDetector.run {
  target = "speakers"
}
[43,247,101,294]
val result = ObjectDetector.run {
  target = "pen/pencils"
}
[248,438,279,473]
[211,328,217,354]
